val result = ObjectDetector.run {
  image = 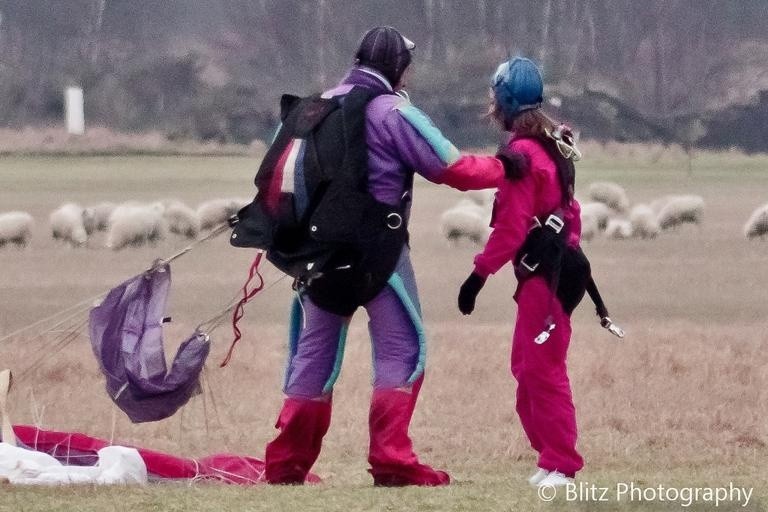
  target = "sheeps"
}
[50,197,254,252]
[1,210,34,246]
[441,189,498,246]
[744,203,768,244]
[574,180,705,243]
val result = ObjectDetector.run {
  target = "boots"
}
[263,396,332,484]
[366,376,450,485]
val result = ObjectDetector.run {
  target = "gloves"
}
[458,270,486,315]
[496,149,530,180]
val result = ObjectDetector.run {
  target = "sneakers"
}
[526,468,575,488]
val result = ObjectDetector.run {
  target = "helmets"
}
[354,25,417,63]
[490,56,544,112]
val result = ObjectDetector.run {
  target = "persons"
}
[265,22,534,488]
[454,54,585,489]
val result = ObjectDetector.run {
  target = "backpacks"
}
[230,87,397,285]
